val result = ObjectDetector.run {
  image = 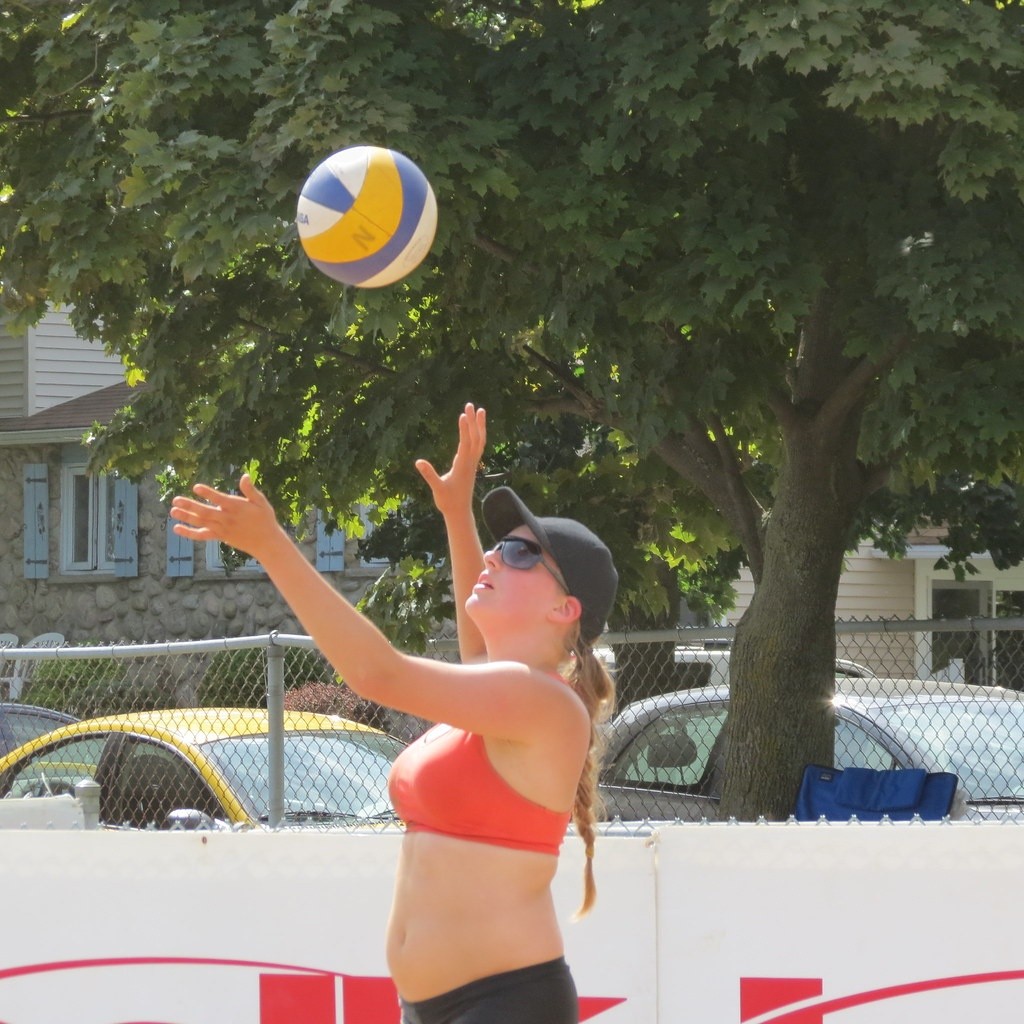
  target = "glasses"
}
[491,536,572,597]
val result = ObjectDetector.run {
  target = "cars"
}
[584,675,1024,822]
[0,706,419,831]
[0,702,83,778]
[575,647,883,786]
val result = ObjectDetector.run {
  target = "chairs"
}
[793,762,960,824]
[0,632,67,701]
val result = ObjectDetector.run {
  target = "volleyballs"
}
[294,144,440,289]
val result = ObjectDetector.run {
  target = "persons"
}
[170,402,616,1024]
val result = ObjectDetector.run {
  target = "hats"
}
[481,487,619,651]
